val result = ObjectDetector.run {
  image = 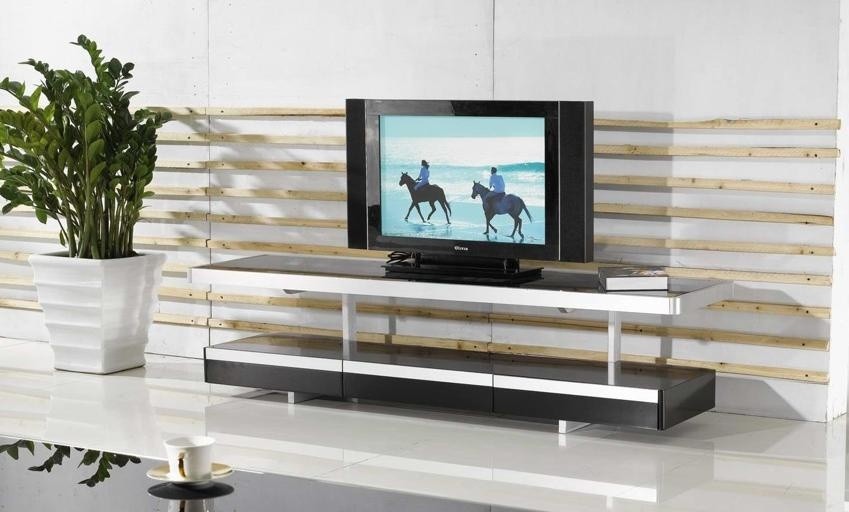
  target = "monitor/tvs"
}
[346,98,594,285]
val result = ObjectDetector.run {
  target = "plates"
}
[146,461,234,485]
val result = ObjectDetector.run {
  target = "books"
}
[598,265,669,291]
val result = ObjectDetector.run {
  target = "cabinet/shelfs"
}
[204,395,714,511]
[186,255,734,433]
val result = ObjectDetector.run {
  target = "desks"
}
[0,435,538,512]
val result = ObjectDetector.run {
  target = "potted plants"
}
[0,34,167,374]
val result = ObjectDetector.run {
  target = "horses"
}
[398,172,451,224]
[471,180,532,239]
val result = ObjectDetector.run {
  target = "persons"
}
[414,159,430,200]
[485,167,505,217]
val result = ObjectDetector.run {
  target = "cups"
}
[162,431,217,480]
